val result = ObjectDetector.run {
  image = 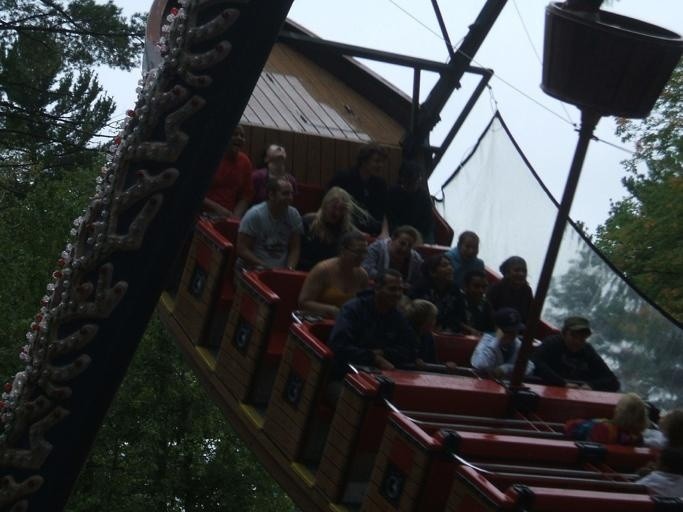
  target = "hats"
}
[495,306,527,333]
[561,316,594,334]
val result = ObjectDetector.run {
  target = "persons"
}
[360,225,425,283]
[470,307,535,380]
[462,271,496,330]
[249,144,295,206]
[321,268,420,405]
[564,392,646,446]
[299,185,378,272]
[379,299,456,371]
[232,171,302,292]
[523,317,620,392]
[444,231,484,288]
[298,230,370,317]
[635,409,683,475]
[486,257,532,324]
[202,125,254,218]
[634,443,683,497]
[640,401,670,450]
[387,163,435,244]
[326,142,389,245]
[407,254,474,331]
